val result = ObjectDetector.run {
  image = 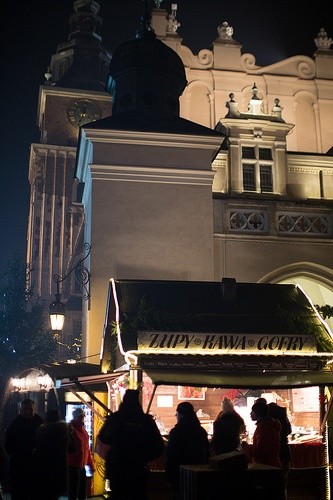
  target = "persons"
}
[32,410,81,500]
[240,398,291,500]
[99,389,164,500]
[3,398,43,500]
[67,407,96,500]
[212,397,246,458]
[164,403,211,500]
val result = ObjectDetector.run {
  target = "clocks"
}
[67,98,102,129]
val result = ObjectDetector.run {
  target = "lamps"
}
[48,242,90,334]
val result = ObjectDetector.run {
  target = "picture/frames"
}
[178,386,205,400]
